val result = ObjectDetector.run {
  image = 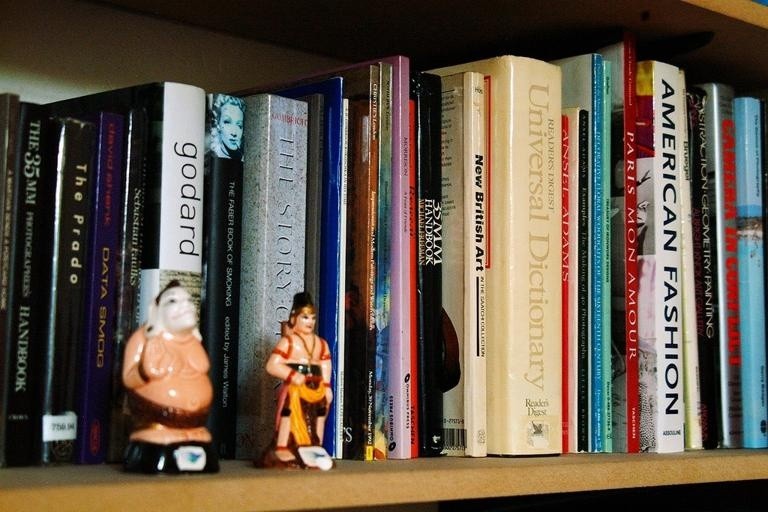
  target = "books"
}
[1,37,768,476]
